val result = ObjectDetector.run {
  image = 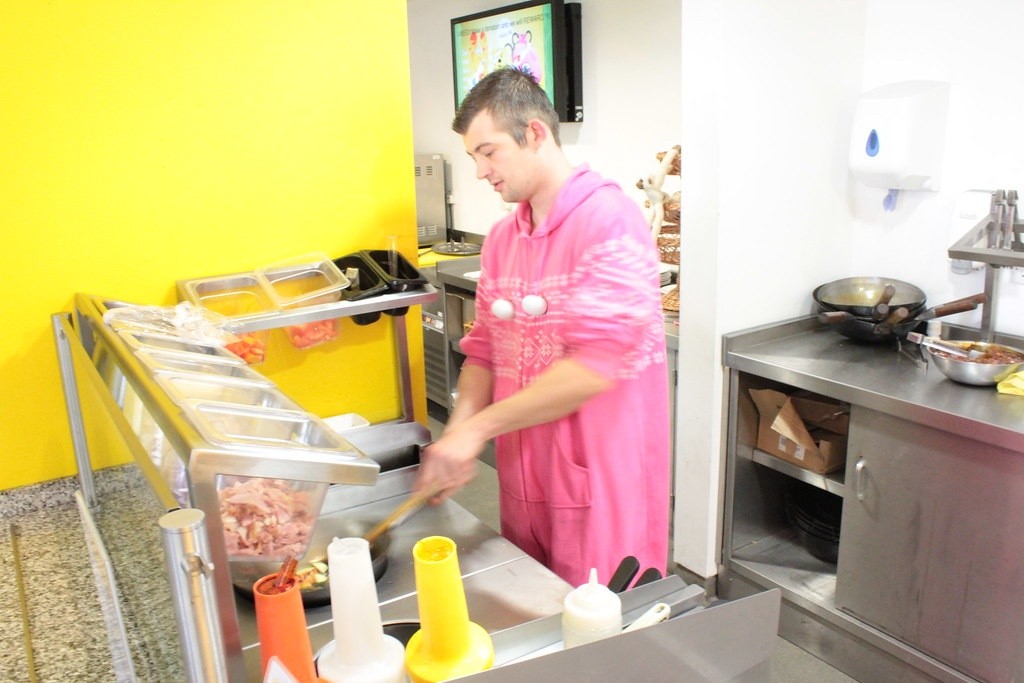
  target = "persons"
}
[416,67,670,590]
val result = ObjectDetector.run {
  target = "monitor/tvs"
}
[451,0,568,123]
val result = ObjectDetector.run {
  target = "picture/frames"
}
[449,0,574,123]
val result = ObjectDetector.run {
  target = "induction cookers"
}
[230,485,578,683]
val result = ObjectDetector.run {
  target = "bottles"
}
[563,568,623,649]
[255,535,494,683]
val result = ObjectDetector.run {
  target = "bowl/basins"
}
[927,340,1024,386]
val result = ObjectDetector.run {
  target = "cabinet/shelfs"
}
[722,368,1024,683]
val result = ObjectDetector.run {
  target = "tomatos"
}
[223,330,264,365]
[284,320,337,349]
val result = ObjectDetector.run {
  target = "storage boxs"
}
[745,386,849,475]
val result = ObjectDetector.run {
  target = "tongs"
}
[906,332,984,361]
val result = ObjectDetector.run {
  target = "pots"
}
[785,492,838,565]
[232,496,434,606]
[812,276,986,342]
